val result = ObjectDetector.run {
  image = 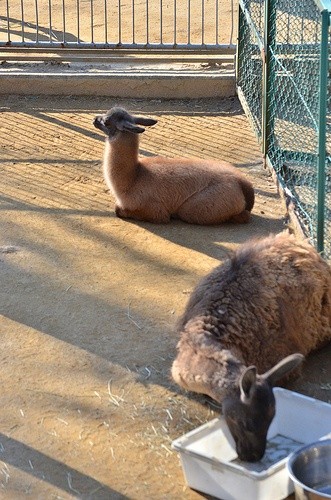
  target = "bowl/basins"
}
[284,438,331,500]
